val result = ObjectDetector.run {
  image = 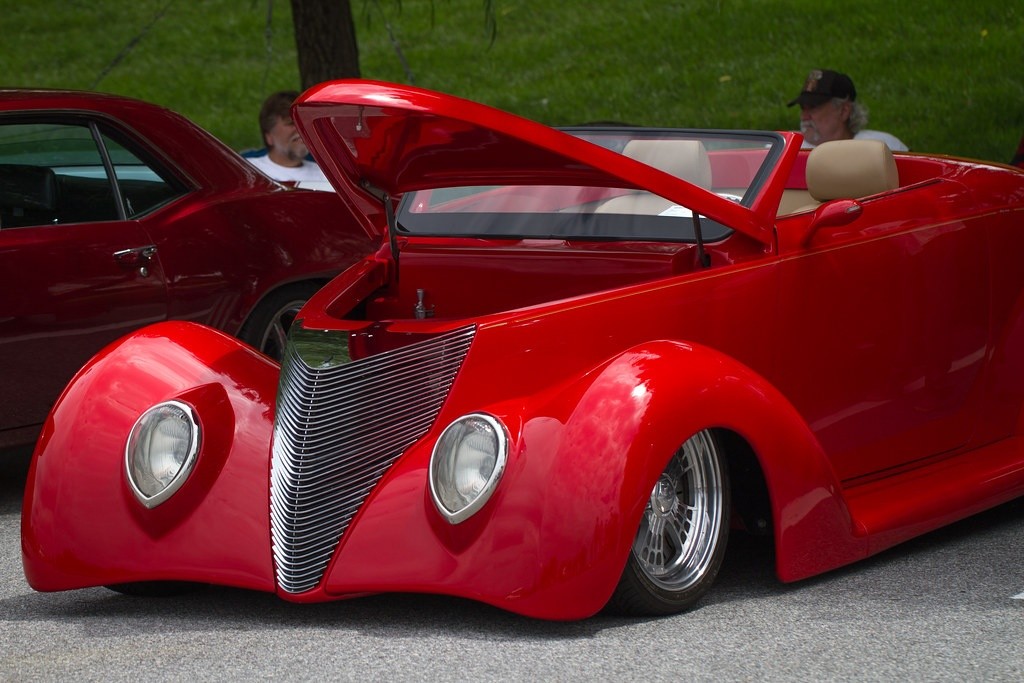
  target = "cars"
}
[20,77,1024,625]
[0,87,396,450]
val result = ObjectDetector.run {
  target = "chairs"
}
[788,139,898,213]
[595,138,711,214]
[0,163,58,225]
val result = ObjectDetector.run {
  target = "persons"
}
[237,90,332,191]
[769,68,910,151]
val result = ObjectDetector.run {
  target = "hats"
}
[785,68,856,107]
